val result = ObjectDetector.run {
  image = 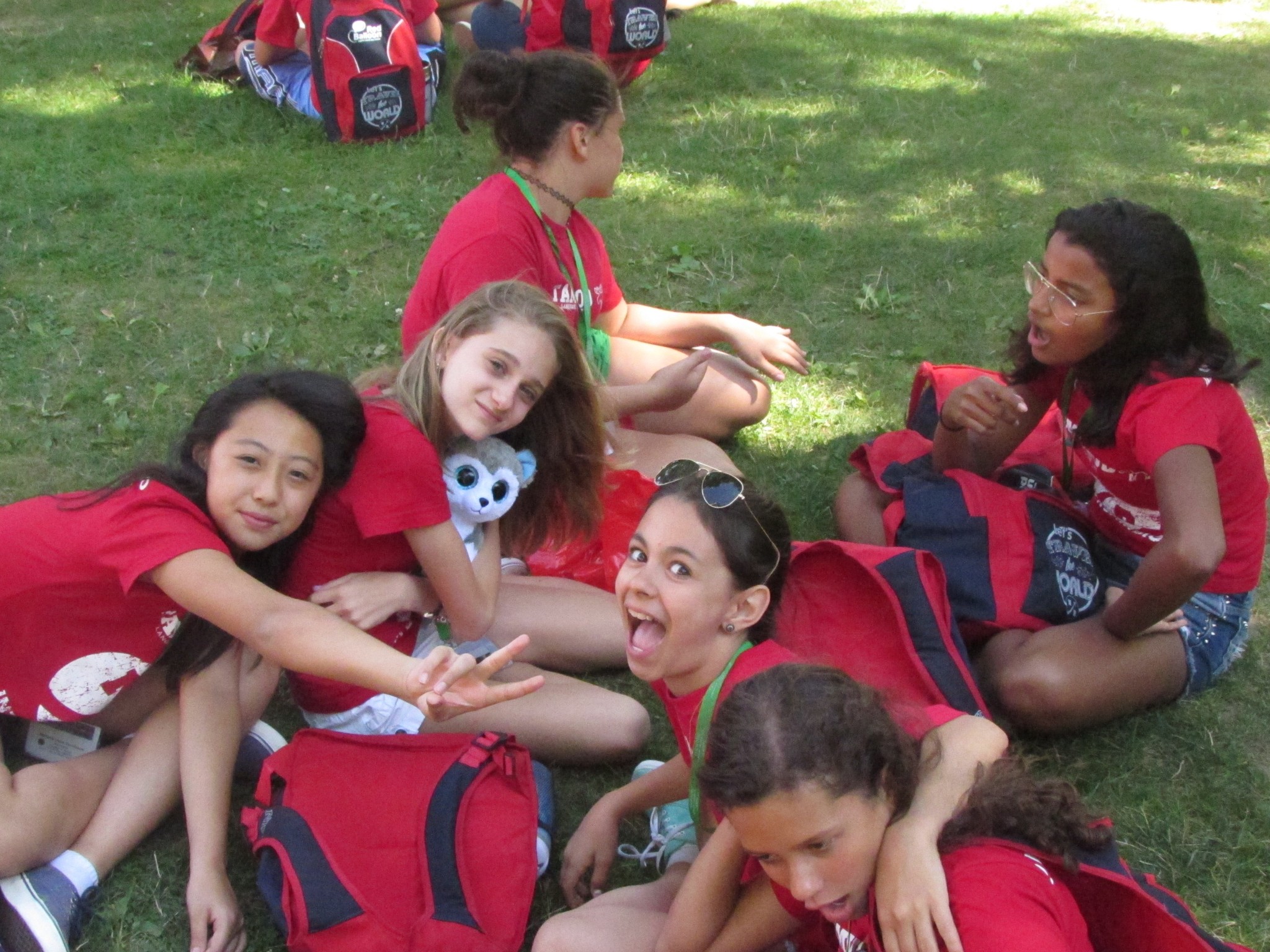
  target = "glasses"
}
[654,457,782,586]
[1020,259,1119,327]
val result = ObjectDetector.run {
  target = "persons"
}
[436,0,671,90]
[398,44,813,484]
[0,368,546,952]
[273,276,653,762]
[530,458,1008,951]
[836,196,1270,726]
[234,0,442,120]
[654,662,1257,951]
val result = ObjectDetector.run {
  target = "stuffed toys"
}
[442,435,537,563]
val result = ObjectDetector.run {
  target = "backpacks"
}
[303,0,433,147]
[769,538,995,749]
[173,0,268,84]
[519,0,672,90]
[238,728,539,952]
[933,805,1257,952]
[846,361,1110,639]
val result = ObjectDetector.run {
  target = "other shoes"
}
[452,18,482,56]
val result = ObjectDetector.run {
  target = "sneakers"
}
[618,759,701,877]
[0,861,97,952]
[232,717,292,783]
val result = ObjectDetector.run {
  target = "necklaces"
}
[690,633,753,853]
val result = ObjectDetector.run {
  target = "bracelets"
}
[392,602,447,647]
[940,401,964,432]
